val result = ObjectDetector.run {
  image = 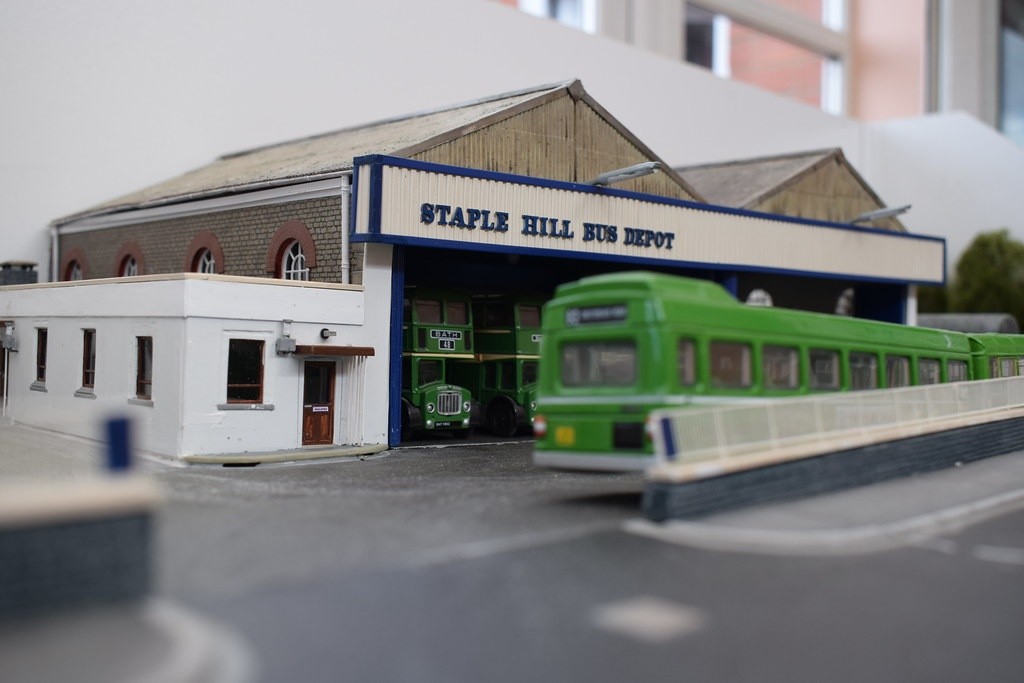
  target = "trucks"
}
[475,285,546,439]
[402,284,483,441]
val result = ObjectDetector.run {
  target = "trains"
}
[532,270,1024,475]
